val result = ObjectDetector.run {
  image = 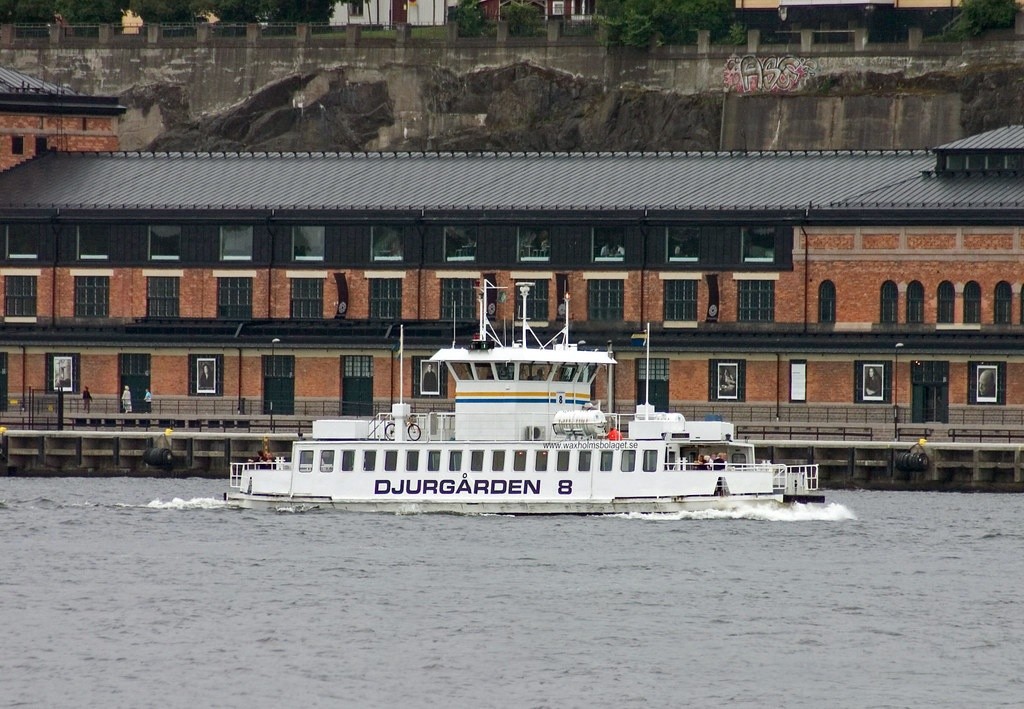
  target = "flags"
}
[630,328,647,339]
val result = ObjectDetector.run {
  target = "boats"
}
[223,279,826,514]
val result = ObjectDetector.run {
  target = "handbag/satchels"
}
[146,396,151,402]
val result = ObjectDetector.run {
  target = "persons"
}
[143,388,152,413]
[81,385,93,413]
[198,363,213,387]
[865,366,882,396]
[721,367,736,388]
[121,384,133,414]
[254,450,276,469]
[422,363,437,391]
[694,452,727,470]
[978,370,994,396]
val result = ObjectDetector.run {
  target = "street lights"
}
[893,343,904,442]
[270,338,280,430]
